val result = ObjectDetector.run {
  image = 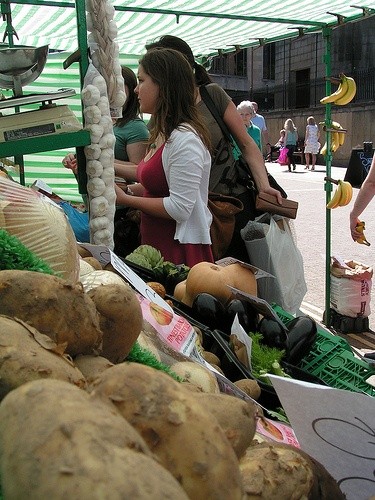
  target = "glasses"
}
[146,36,162,45]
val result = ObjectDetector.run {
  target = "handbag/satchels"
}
[207,190,243,260]
[241,212,310,316]
[236,155,287,206]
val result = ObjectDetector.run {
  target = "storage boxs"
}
[258,302,375,397]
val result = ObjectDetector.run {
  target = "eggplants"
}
[190,292,327,386]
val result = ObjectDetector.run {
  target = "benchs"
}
[270,146,306,164]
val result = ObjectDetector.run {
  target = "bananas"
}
[318,121,344,155]
[320,73,357,105]
[326,179,353,209]
[355,222,370,246]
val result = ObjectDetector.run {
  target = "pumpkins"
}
[174,260,257,306]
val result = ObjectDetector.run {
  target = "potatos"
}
[234,440,349,500]
[0,270,262,500]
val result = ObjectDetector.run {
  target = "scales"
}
[0,44,83,143]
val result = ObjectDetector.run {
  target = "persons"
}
[350,151,375,242]
[267,129,285,163]
[61,66,151,261]
[229,100,262,160]
[112,46,213,271]
[303,115,321,171]
[251,101,271,156]
[144,34,282,263]
[283,119,299,172]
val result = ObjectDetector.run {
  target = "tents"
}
[0,0,375,326]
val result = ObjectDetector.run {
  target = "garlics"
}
[85,0,127,109]
[81,74,117,252]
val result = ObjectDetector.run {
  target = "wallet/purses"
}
[256,192,299,219]
